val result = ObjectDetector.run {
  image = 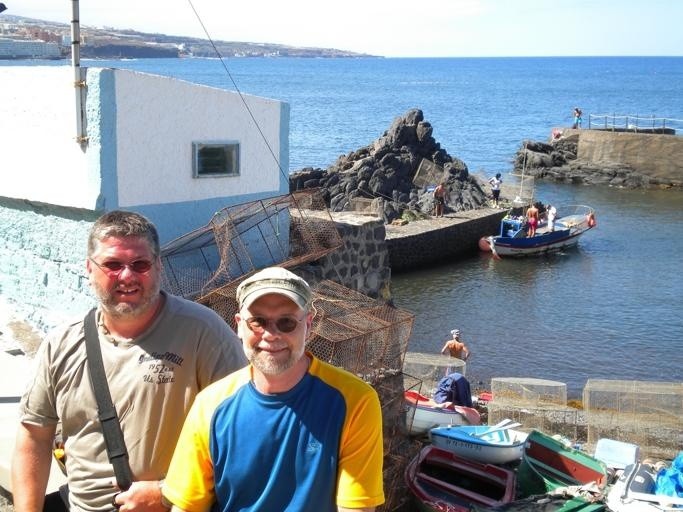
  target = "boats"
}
[400,390,472,435]
[429,423,529,465]
[519,430,606,496]
[486,204,597,259]
[405,446,516,512]
[603,454,682,512]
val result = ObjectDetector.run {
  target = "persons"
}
[546,204,556,233]
[525,202,538,237]
[431,182,446,219]
[440,328,470,377]
[488,172,503,209]
[6,208,249,511]
[158,266,387,512]
[573,108,582,130]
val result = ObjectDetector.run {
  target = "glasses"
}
[245,316,307,333]
[90,256,157,273]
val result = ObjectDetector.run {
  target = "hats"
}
[235,266,312,314]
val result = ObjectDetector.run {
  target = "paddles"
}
[475,418,521,438]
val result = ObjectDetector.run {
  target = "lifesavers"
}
[587,214,595,227]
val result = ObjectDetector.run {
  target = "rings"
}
[111,493,118,508]
